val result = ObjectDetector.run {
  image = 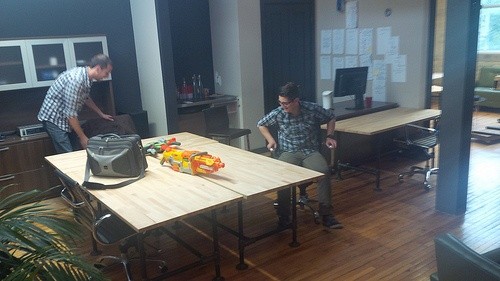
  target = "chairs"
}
[428,232,500,281]
[393,91,443,191]
[202,105,251,151]
[266,126,338,224]
[55,169,169,281]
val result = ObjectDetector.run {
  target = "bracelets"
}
[326,135,335,140]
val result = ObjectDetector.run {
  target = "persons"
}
[38,53,114,155]
[257,82,344,230]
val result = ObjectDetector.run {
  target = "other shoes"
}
[322,215,344,228]
[278,214,291,227]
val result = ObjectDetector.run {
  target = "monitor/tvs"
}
[333,66,368,109]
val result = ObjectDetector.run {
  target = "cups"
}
[365,97,372,108]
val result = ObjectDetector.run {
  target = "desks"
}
[140,131,325,270]
[44,149,243,281]
[321,107,442,191]
[323,98,397,170]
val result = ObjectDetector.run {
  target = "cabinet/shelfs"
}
[0,140,65,210]
[0,34,113,92]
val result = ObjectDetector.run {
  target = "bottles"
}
[179,73,205,99]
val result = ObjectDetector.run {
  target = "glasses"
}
[277,97,297,107]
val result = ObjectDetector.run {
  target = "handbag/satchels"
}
[81,133,147,189]
